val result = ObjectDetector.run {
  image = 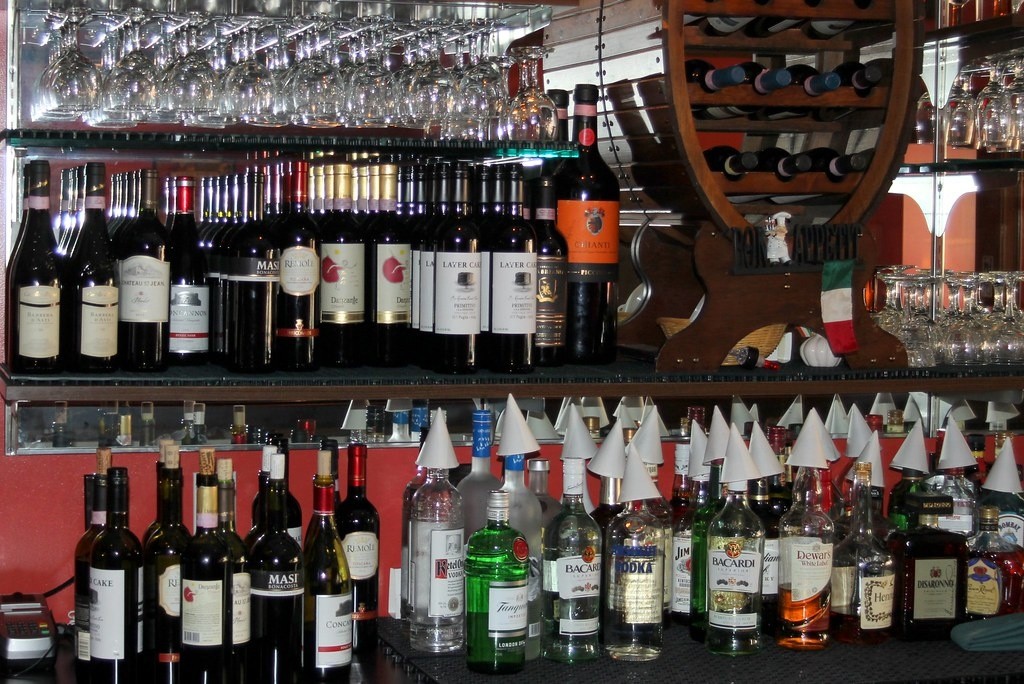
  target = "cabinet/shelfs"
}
[0,12,1024,457]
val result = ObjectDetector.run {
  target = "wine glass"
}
[871,264,1024,367]
[918,60,1024,152]
[32,0,559,141]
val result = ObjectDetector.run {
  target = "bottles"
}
[337,444,379,651]
[143,439,173,546]
[9,83,616,370]
[401,410,1024,674]
[266,437,302,547]
[316,440,340,508]
[90,466,143,684]
[702,145,866,205]
[52,400,447,445]
[299,451,353,684]
[685,59,745,111]
[583,407,705,439]
[812,62,882,122]
[251,453,300,684]
[181,448,232,684]
[696,62,791,119]
[753,65,841,119]
[75,448,111,666]
[144,444,190,684]
[684,0,874,40]
[217,458,251,684]
[245,445,277,547]
